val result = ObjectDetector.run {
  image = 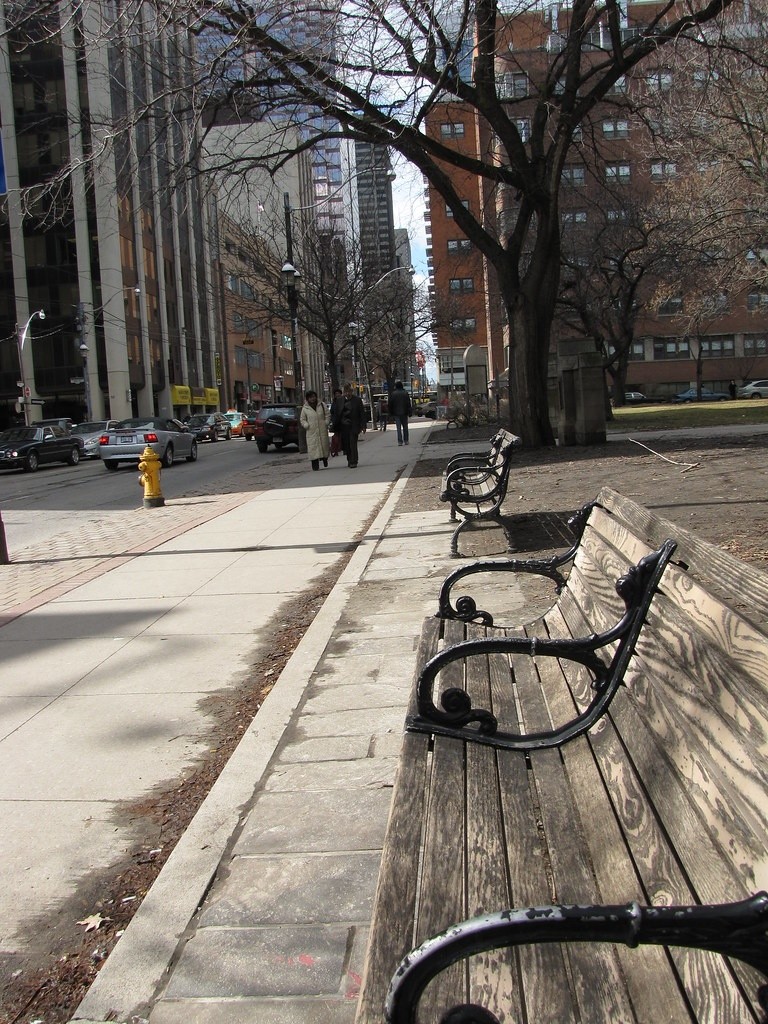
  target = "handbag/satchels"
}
[330,432,342,453]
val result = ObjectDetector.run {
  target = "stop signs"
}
[25,387,31,397]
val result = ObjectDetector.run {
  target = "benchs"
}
[357,484,768,1024]
[439,428,523,558]
[445,407,468,429]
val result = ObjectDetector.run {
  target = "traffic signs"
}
[31,399,46,405]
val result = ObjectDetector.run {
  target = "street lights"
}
[281,262,306,454]
[14,308,45,427]
[348,322,362,396]
[282,164,397,451]
[410,372,415,397]
[75,286,141,421]
[245,344,282,409]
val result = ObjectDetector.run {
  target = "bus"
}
[407,391,437,405]
[381,382,411,400]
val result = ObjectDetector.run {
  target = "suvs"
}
[254,403,299,453]
[186,411,231,442]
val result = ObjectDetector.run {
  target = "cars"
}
[610,391,645,406]
[99,417,197,470]
[223,394,388,440]
[0,406,126,472]
[736,379,768,399]
[422,401,436,419]
[671,387,730,404]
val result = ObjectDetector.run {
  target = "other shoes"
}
[398,440,403,445]
[349,463,357,468]
[324,460,328,467]
[314,465,319,470]
[405,442,409,445]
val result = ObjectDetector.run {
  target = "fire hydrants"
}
[137,444,165,509]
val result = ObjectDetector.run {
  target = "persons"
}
[300,391,331,470]
[330,390,346,455]
[729,380,737,399]
[335,384,366,468]
[377,396,387,431]
[389,382,412,446]
